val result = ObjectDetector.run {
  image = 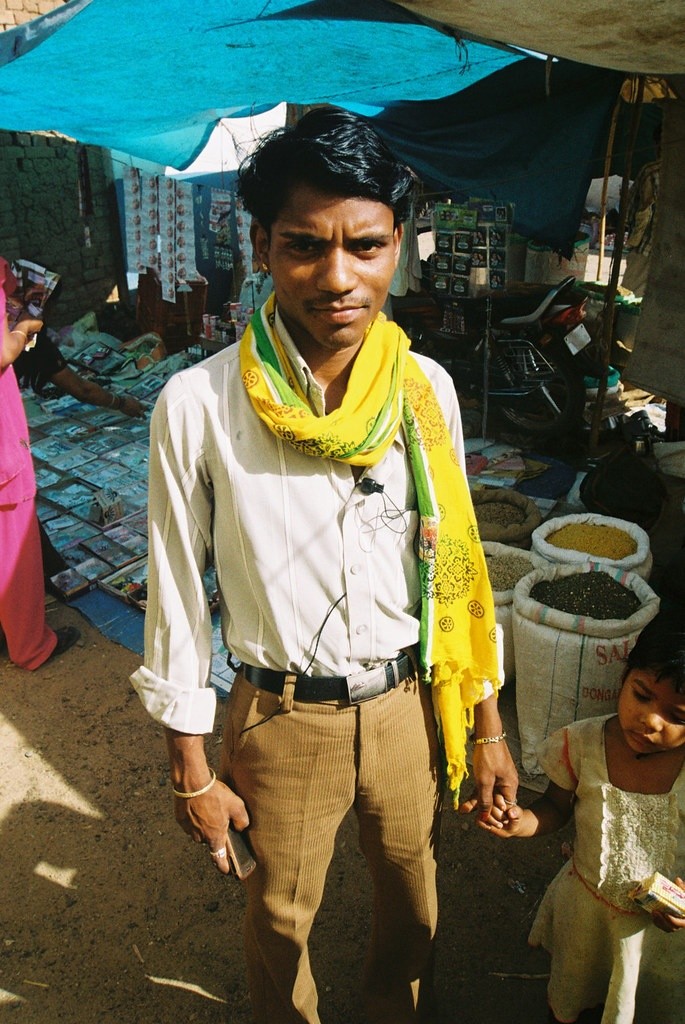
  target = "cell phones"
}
[224,819,258,883]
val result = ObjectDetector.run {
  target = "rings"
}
[504,800,517,806]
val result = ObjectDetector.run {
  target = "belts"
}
[243,651,422,705]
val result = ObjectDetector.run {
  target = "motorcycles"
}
[391,274,603,438]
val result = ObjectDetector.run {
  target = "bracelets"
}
[472,731,509,745]
[109,393,116,406]
[116,394,124,410]
[172,769,216,799]
[11,331,29,342]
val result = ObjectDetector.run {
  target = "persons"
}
[473,605,685,1024]
[10,261,152,421]
[1,256,79,671]
[129,105,520,1024]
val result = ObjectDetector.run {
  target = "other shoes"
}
[48,626,80,659]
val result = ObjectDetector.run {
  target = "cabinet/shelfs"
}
[134,268,208,346]
